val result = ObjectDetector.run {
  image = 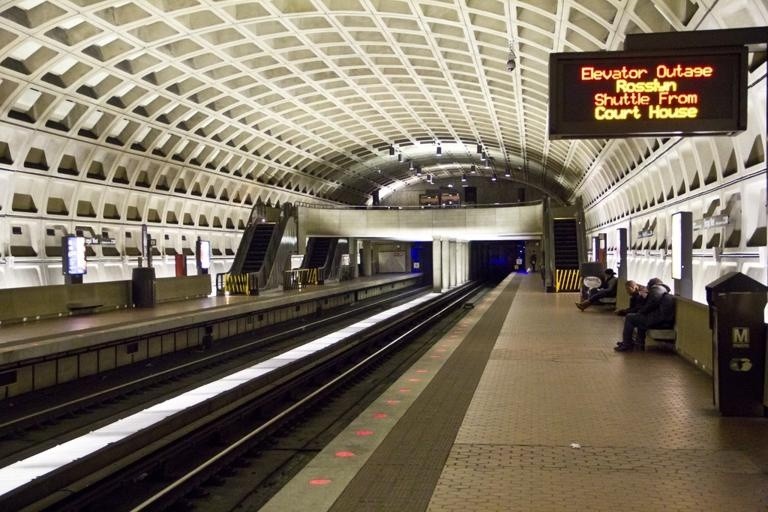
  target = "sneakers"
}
[614,341,635,352]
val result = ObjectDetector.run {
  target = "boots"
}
[574,300,592,311]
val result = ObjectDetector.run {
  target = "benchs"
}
[631,324,678,343]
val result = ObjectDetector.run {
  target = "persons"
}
[618,281,647,315]
[530,250,537,272]
[614,278,675,352]
[577,269,617,311]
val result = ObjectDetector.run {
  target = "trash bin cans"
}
[132,267,156,308]
[706,272,768,417]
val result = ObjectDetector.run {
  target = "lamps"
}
[506,44,518,73]
[384,138,515,192]
